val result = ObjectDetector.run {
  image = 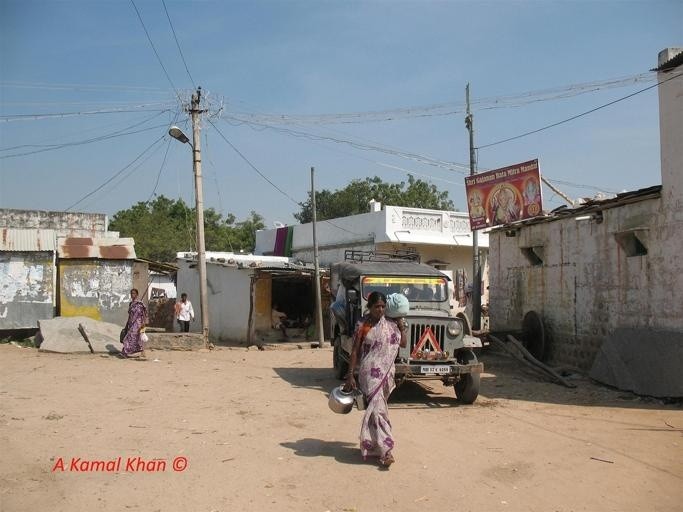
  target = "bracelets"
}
[398,318,408,332]
[347,368,354,375]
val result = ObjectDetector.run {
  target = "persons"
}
[271,302,289,338]
[345,292,409,467]
[174,293,195,332]
[120,288,148,361]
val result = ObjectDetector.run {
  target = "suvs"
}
[328,250,483,404]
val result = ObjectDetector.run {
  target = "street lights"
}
[165,124,208,344]
